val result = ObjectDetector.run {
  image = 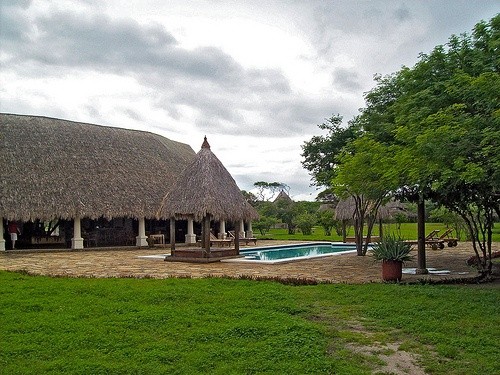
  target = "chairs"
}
[200,228,234,247]
[401,229,459,250]
[228,231,257,245]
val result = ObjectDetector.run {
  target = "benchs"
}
[346,237,366,243]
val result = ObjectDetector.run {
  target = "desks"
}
[71,228,166,248]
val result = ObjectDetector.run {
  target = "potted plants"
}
[367,230,416,282]
